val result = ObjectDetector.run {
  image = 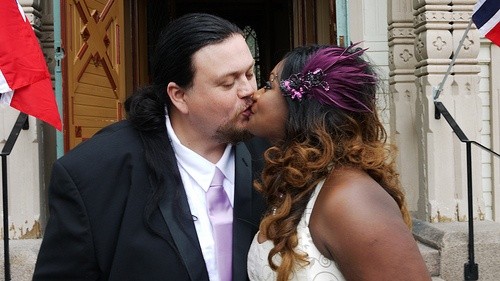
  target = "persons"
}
[30,14,279,281]
[246,40,430,281]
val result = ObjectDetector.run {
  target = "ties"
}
[206,167,234,281]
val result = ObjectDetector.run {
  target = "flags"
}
[472,1,500,47]
[0,0,63,133]
